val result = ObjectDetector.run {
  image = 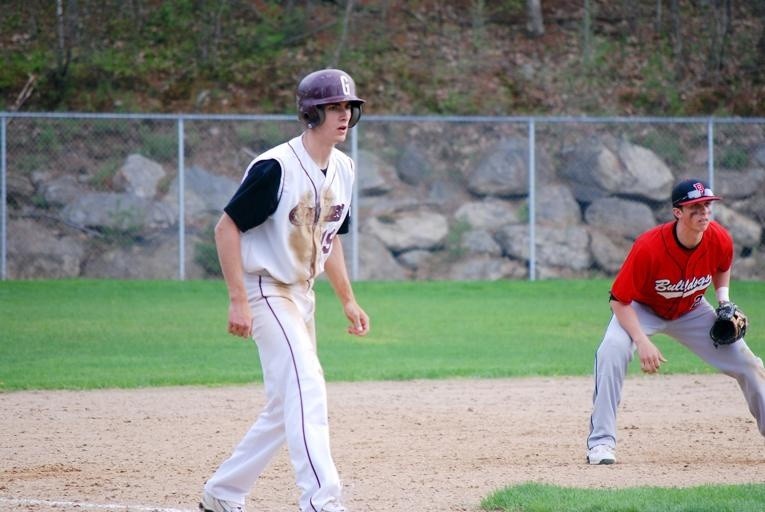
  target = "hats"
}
[671,179,722,205]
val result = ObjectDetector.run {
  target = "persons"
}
[582,182,765,466]
[197,68,371,512]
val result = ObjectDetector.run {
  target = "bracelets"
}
[715,282,732,305]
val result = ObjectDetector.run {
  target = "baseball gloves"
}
[709,301,747,347]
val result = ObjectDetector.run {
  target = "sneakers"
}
[299,500,348,512]
[198,491,245,512]
[587,444,616,465]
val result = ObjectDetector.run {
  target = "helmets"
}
[296,69,367,130]
